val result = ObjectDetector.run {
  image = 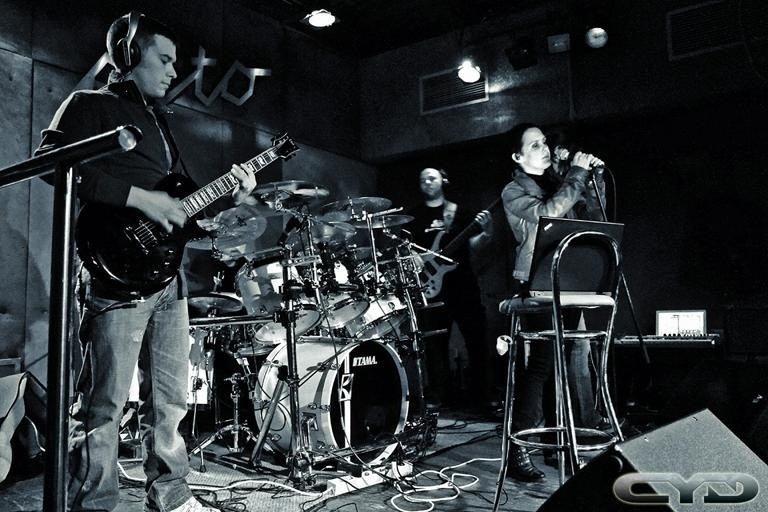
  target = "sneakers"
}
[143,496,221,512]
[491,401,505,416]
[426,400,442,408]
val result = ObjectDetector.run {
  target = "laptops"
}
[485,216,625,298]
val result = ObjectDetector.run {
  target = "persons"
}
[35,9,262,510]
[188,248,250,292]
[399,164,512,424]
[500,119,612,481]
[563,306,627,446]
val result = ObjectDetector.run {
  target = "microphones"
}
[553,144,606,176]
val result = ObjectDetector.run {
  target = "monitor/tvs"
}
[654,310,709,338]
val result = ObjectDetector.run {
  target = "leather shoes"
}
[595,417,625,433]
[507,447,545,481]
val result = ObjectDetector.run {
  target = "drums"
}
[253,336,409,467]
[345,266,411,343]
[321,255,369,329]
[233,258,321,344]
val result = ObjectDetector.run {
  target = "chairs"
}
[491,213,624,511]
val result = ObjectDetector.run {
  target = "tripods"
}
[186,391,274,461]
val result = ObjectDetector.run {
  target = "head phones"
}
[439,169,451,190]
[114,11,140,73]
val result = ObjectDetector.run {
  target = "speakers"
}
[535,407,768,512]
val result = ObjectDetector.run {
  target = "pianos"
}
[612,329,721,354]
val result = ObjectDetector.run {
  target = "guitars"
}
[78,130,301,305]
[408,194,506,299]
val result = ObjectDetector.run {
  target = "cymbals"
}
[351,212,416,230]
[244,179,330,200]
[319,194,394,217]
